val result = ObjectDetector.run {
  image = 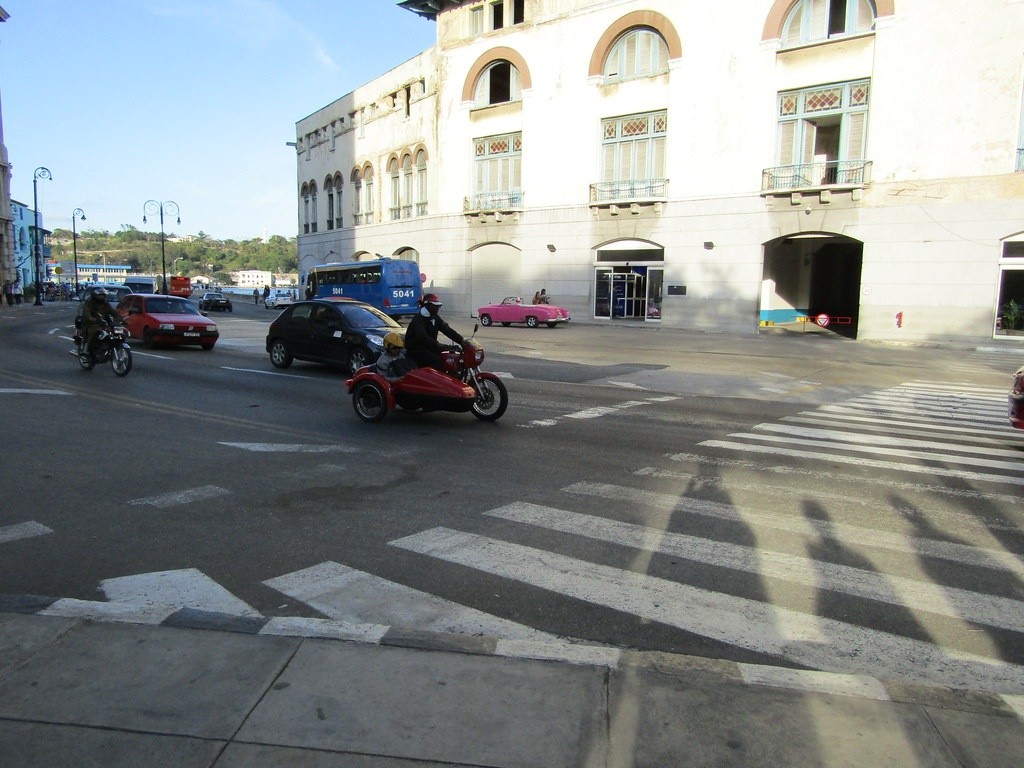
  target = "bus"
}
[165,276,191,298]
[301,257,422,321]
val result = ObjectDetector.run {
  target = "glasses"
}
[390,346,399,350]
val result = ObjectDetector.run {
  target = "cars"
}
[198,293,233,312]
[43,281,76,296]
[78,284,139,316]
[596,297,623,314]
[265,294,295,309]
[266,298,407,375]
[115,294,219,350]
[1008,366,1024,429]
[477,297,572,328]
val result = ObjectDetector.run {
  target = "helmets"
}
[382,332,405,352]
[90,287,107,303]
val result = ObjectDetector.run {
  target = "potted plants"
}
[1001,299,1019,336]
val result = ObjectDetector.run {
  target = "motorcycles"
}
[344,323,509,421]
[69,315,133,376]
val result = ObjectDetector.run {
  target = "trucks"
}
[121,276,160,295]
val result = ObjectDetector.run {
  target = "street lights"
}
[33,167,53,306]
[100,254,106,284]
[142,200,181,295]
[72,208,86,296]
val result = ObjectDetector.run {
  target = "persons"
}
[404,294,464,371]
[264,285,269,297]
[81,286,124,354]
[3,280,23,305]
[532,288,549,305]
[377,332,406,381]
[254,289,259,304]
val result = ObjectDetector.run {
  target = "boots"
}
[83,343,90,355]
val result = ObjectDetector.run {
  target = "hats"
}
[418,293,442,307]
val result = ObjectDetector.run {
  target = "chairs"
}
[367,365,379,373]
[294,316,306,320]
